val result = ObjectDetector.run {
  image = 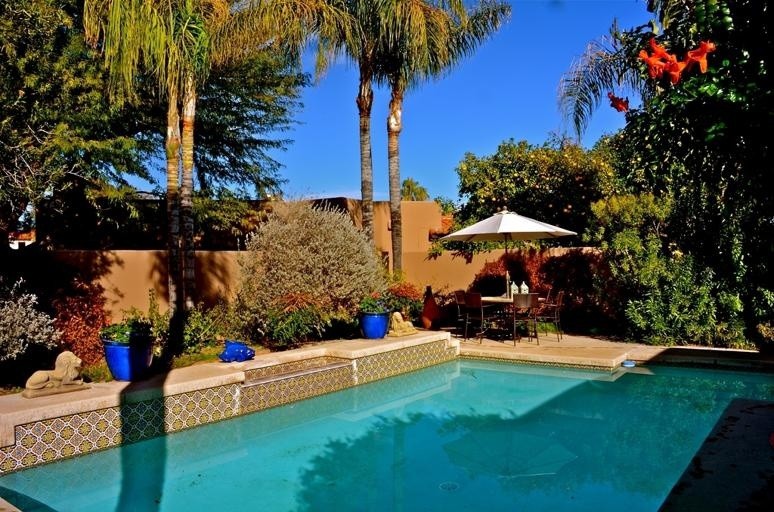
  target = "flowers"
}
[96,306,151,343]
[358,290,388,314]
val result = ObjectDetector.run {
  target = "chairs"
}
[455,287,564,346]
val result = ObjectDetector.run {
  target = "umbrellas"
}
[438,206,577,299]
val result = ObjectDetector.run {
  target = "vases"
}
[102,339,153,381]
[360,313,389,340]
